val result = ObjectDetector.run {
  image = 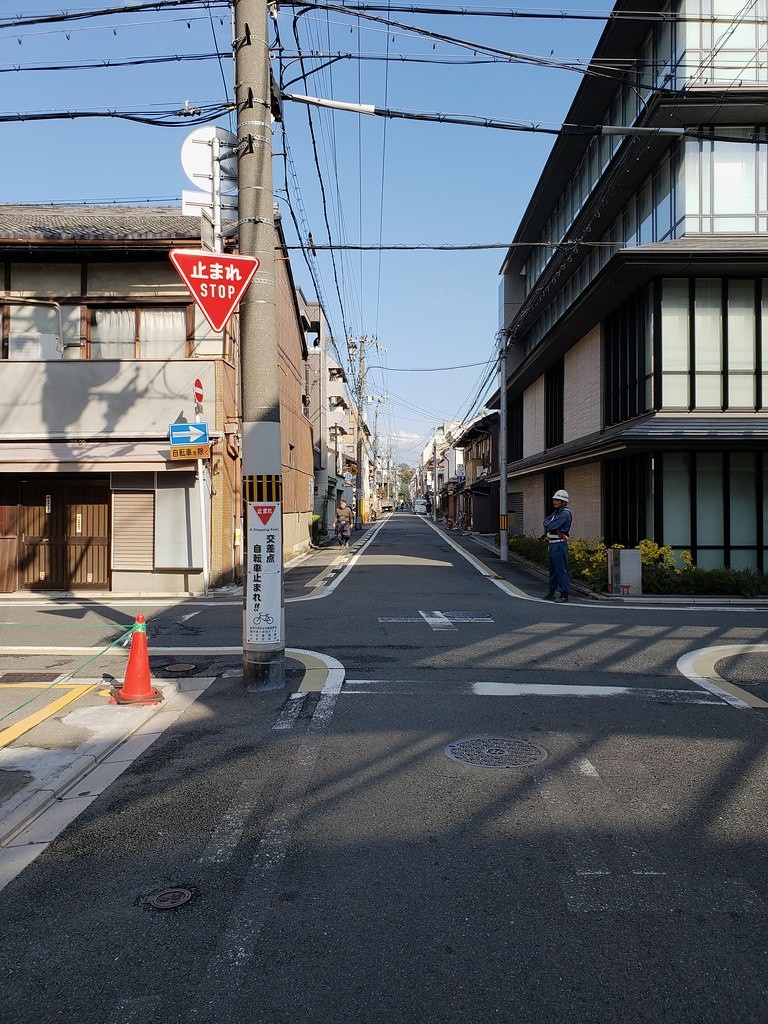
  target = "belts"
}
[547,533,562,538]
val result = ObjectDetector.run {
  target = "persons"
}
[400,502,404,511]
[332,500,353,549]
[542,489,573,603]
[426,501,433,518]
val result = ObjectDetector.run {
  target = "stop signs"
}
[166,248,261,335]
[194,377,205,403]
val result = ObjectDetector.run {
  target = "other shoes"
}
[542,593,555,601]
[554,594,568,603]
[341,544,345,549]
[345,542,349,548]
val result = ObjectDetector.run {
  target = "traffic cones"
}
[114,614,158,702]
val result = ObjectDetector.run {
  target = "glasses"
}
[553,499,560,502]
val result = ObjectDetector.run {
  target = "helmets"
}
[552,490,569,502]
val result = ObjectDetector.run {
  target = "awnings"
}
[453,412,498,451]
